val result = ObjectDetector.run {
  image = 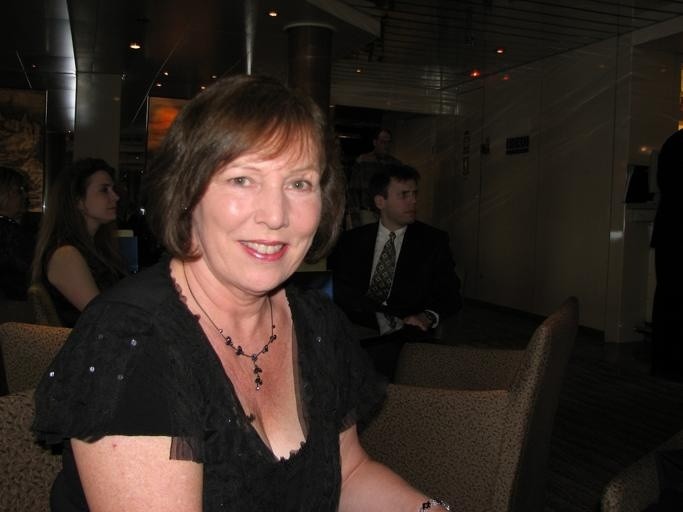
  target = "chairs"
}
[1,281,75,512]
[601,430,683,512]
[358,296,580,511]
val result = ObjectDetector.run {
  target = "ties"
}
[371,232,396,304]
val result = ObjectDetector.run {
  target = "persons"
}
[286,164,351,318]
[37,73,459,511]
[321,160,462,365]
[650,127,682,379]
[0,167,31,302]
[38,155,129,328]
[350,127,402,204]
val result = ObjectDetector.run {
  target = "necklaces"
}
[180,257,277,389]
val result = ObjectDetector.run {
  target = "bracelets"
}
[425,309,436,332]
[415,497,451,511]
